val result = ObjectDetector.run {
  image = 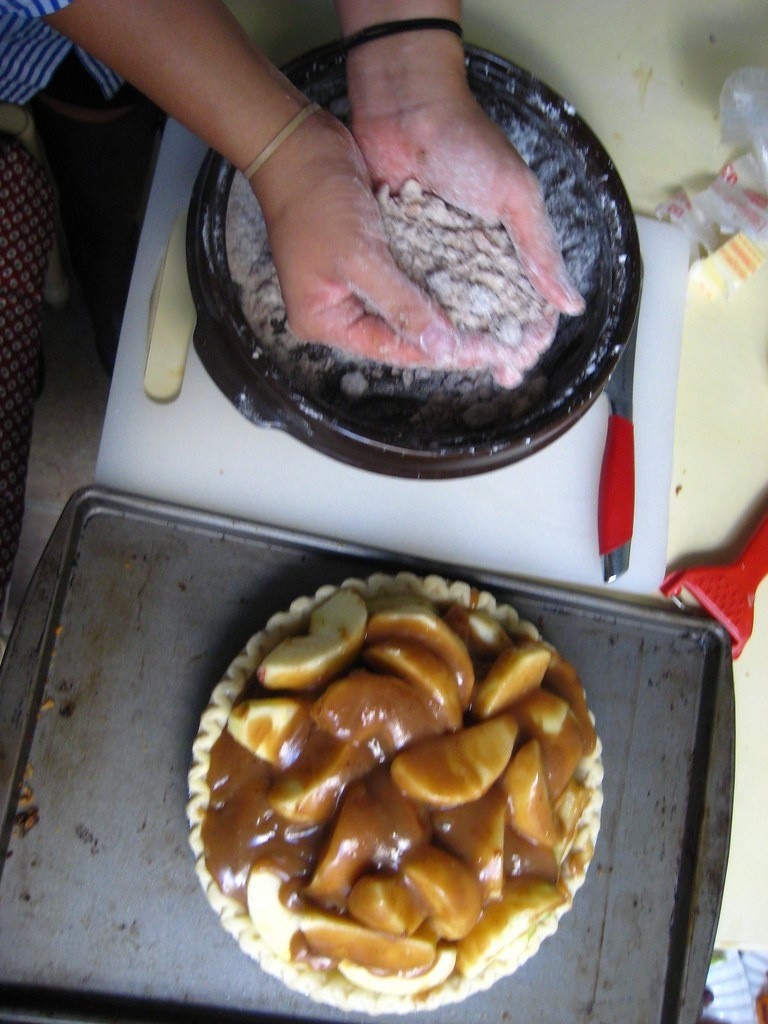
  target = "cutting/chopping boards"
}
[93,115,692,593]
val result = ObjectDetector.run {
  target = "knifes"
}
[597,308,637,585]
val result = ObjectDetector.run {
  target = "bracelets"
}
[243,100,322,181]
[340,17,463,56]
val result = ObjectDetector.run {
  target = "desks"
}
[92,0,768,953]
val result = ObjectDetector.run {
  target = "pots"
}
[186,35,644,481]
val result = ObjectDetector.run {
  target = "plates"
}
[186,566,605,1015]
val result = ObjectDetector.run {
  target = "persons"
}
[0,0,587,390]
